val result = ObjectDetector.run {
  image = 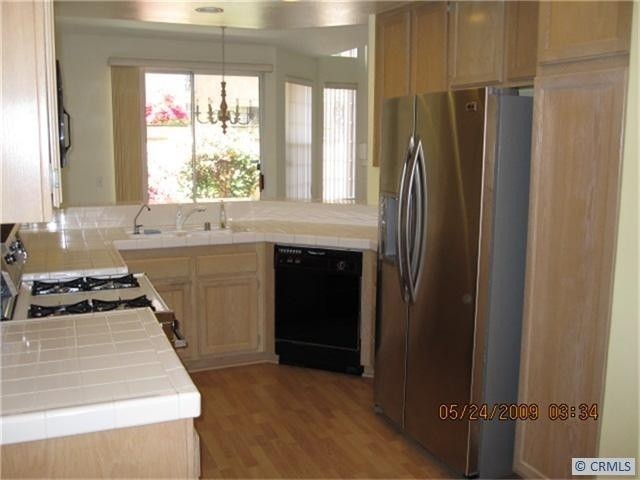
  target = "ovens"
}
[157,320,189,348]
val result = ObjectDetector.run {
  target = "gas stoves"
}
[13,272,178,325]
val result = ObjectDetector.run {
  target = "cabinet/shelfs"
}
[0,0,65,226]
[0,419,202,480]
[370,1,446,167]
[120,243,275,373]
[438,1,537,89]
[513,1,630,479]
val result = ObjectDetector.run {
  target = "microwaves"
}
[56,60,73,167]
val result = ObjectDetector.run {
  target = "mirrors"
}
[57,16,369,207]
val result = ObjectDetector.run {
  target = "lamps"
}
[192,26,257,133]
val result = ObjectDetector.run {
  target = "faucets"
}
[134,204,151,232]
[177,207,205,228]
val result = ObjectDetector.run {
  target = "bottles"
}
[219,200,226,227]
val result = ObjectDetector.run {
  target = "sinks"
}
[142,228,161,235]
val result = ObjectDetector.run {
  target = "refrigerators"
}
[372,83,536,479]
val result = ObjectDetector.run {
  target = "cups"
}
[205,222,210,230]
